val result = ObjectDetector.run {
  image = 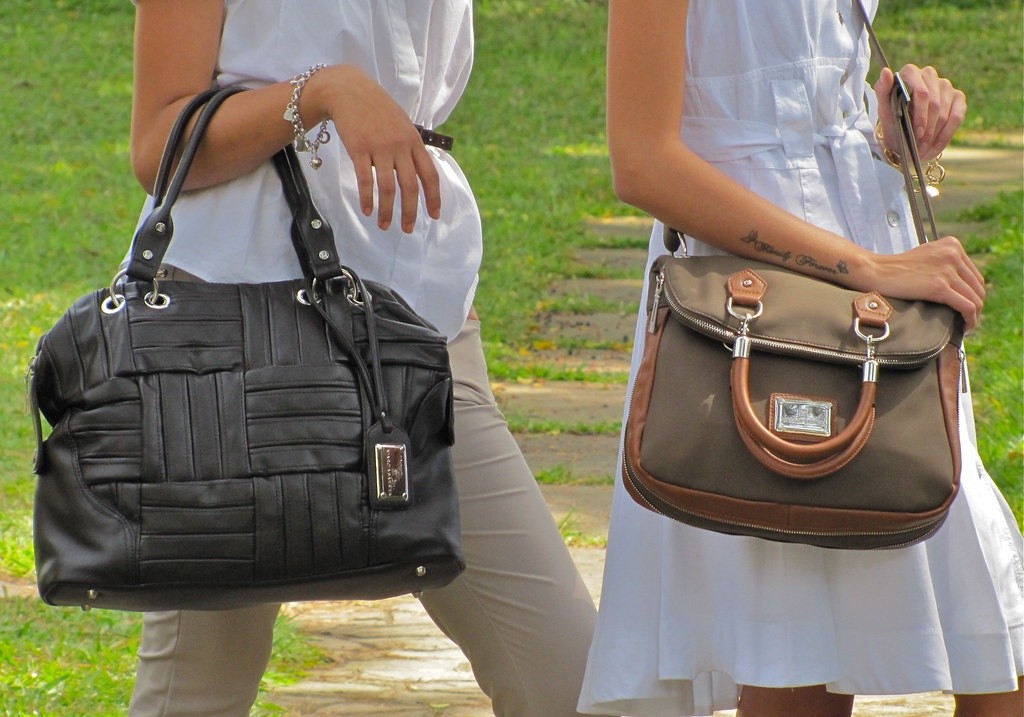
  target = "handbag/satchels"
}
[30,87,467,612]
[620,253,968,553]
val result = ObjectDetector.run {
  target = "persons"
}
[118,0,598,717]
[576,0,1024,717]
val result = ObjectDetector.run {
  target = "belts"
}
[412,124,455,150]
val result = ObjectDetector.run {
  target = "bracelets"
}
[874,119,946,198]
[283,63,331,171]
